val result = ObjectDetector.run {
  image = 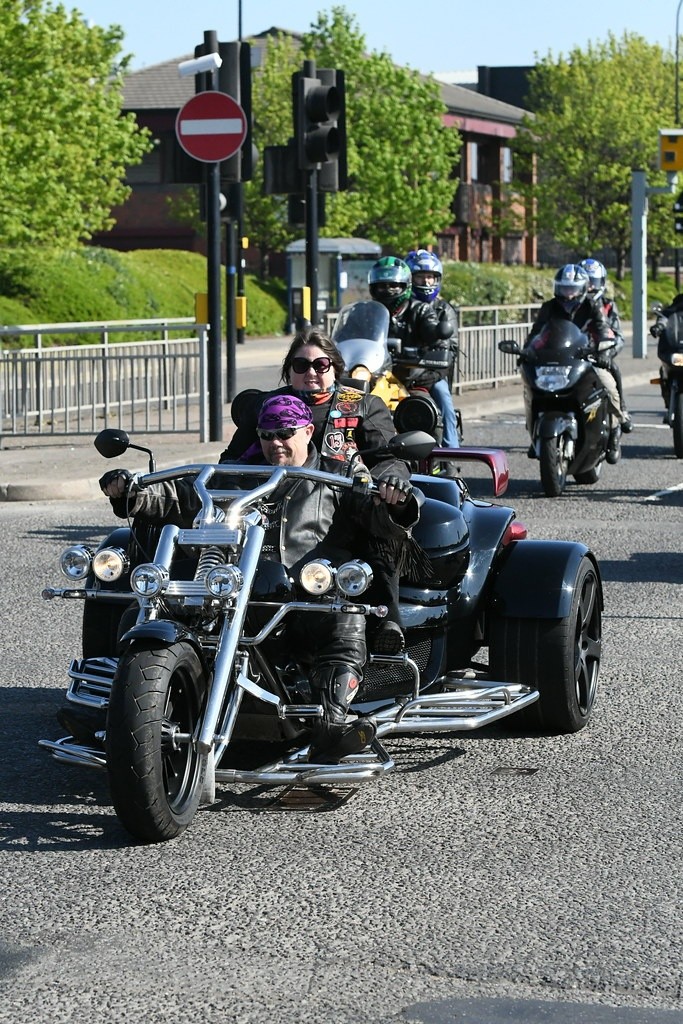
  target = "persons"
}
[338,249,461,496]
[649,293,683,424]
[219,329,412,670]
[56,397,424,764]
[518,259,633,464]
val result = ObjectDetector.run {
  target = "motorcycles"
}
[297,300,463,486]
[498,319,614,497]
[44,428,607,841]
[650,297,683,459]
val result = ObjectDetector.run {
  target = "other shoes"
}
[663,411,675,425]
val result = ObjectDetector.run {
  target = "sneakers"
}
[368,620,405,670]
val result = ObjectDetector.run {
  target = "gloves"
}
[415,371,442,388]
[650,324,665,338]
[430,346,446,352]
[596,353,611,369]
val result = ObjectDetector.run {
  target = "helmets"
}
[403,249,443,302]
[602,279,614,302]
[553,264,589,308]
[367,256,412,313]
[576,259,607,301]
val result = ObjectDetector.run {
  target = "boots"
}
[307,665,376,765]
[56,612,134,747]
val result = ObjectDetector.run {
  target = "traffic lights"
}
[299,76,339,170]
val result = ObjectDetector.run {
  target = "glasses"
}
[290,357,332,374]
[256,426,307,441]
[376,281,401,289]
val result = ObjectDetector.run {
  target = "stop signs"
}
[176,90,247,163]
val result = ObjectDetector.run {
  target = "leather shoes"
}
[606,427,622,464]
[444,462,457,477]
[527,440,536,459]
[620,410,633,434]
